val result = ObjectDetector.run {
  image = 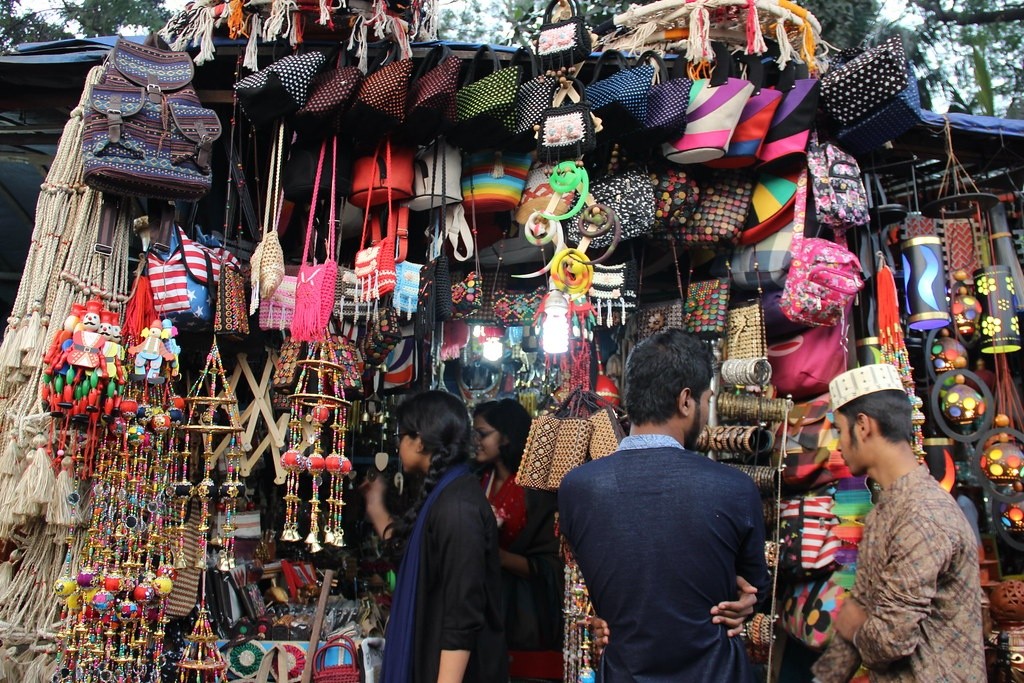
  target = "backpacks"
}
[82,35,221,203]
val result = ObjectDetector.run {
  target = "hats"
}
[828,364,907,413]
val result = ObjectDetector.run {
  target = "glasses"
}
[390,431,419,446]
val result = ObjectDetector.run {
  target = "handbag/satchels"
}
[147,33,915,683]
[536,0,591,71]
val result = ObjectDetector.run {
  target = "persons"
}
[42,301,131,427]
[361,388,509,683]
[556,326,775,683]
[808,361,988,683]
[472,398,558,683]
